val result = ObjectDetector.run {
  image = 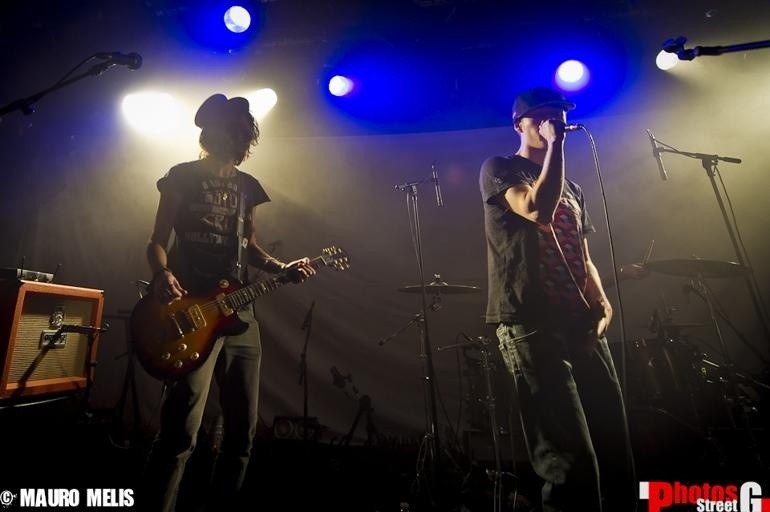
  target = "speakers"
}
[273,415,321,442]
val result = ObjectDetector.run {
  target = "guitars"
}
[128,246,351,383]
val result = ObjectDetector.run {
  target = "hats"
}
[513,88,576,122]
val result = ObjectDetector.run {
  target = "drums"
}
[608,335,697,434]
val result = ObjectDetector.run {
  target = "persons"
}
[600,263,646,289]
[478,88,638,512]
[135,93,316,510]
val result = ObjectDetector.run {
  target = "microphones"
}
[560,124,583,131]
[431,159,444,207]
[96,52,143,68]
[643,128,670,181]
[301,298,319,332]
[60,322,112,335]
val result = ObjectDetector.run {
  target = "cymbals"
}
[642,258,749,277]
[395,284,482,294]
